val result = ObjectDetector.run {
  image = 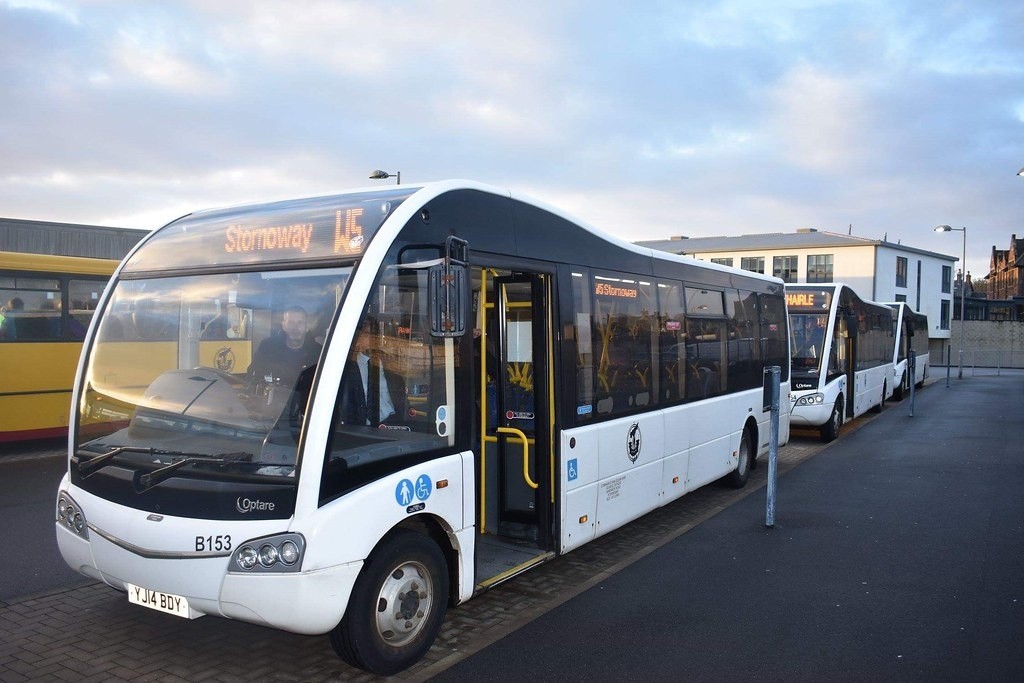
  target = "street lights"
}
[934,224,967,380]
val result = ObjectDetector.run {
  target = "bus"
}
[55,178,792,675]
[784,282,894,442]
[877,302,929,400]
[0,251,253,445]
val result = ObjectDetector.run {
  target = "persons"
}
[286,310,412,458]
[1,293,99,311]
[801,317,835,368]
[195,268,275,376]
[247,292,327,415]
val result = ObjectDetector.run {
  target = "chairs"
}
[594,356,722,414]
